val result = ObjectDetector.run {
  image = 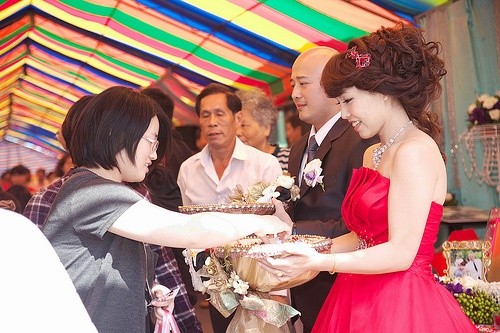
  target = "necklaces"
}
[373,120,413,171]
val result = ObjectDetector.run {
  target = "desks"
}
[439,204,491,237]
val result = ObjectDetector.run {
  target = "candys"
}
[232,231,315,248]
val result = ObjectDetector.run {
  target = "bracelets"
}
[292,222,297,235]
[328,253,337,275]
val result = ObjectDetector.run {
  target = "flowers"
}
[229,171,302,205]
[204,233,308,295]
[302,159,324,188]
[466,91,500,126]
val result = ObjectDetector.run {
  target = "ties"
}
[305,134,318,164]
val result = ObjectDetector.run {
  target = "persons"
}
[271,47,381,333]
[234,87,291,176]
[259,22,482,333]
[41,85,292,333]
[141,86,199,308]
[177,83,284,333]
[0,153,75,212]
[25,95,205,333]
[284,110,311,147]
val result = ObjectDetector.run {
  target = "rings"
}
[276,270,285,278]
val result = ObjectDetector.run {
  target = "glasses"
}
[143,135,159,150]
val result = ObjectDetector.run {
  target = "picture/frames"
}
[442,240,493,284]
